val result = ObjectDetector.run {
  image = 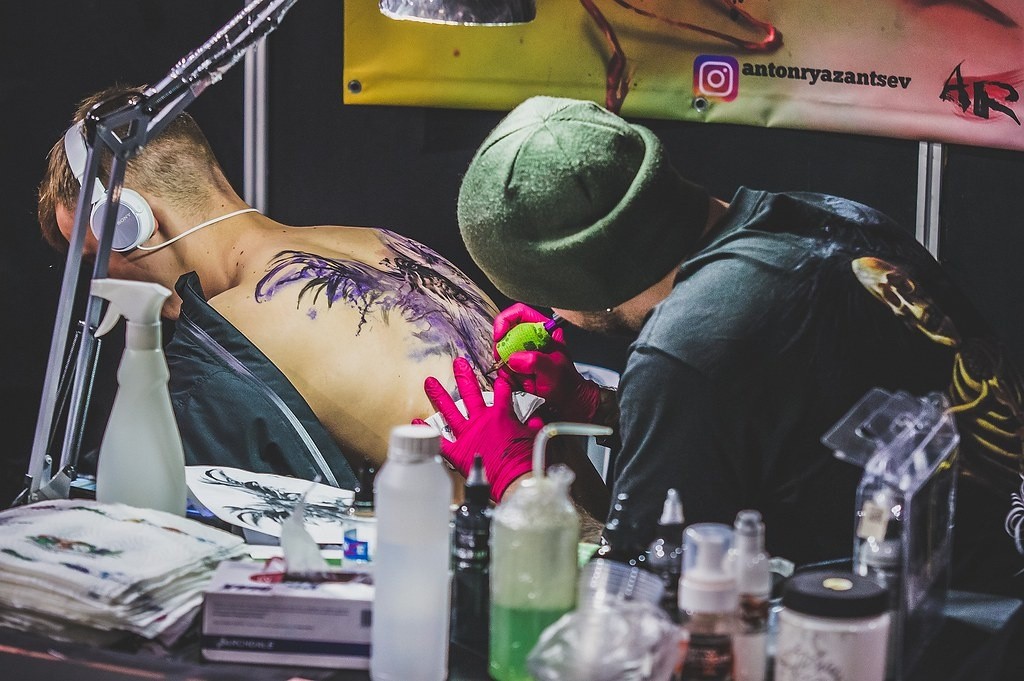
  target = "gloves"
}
[494,303,599,426]
[412,357,546,506]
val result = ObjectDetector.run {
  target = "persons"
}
[412,96,1024,602]
[38,83,504,511]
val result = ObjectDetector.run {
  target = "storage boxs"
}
[198,584,374,675]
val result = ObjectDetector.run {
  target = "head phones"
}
[64,119,155,252]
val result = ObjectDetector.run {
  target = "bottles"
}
[676,522,739,680]
[486,478,581,680]
[369,425,453,681]
[344,455,376,574]
[448,453,498,659]
[773,572,889,680]
[723,510,771,681]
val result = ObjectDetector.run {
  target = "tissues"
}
[201,475,374,671]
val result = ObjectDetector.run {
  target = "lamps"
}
[8,0,542,508]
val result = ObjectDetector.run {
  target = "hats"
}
[459,94,708,312]
